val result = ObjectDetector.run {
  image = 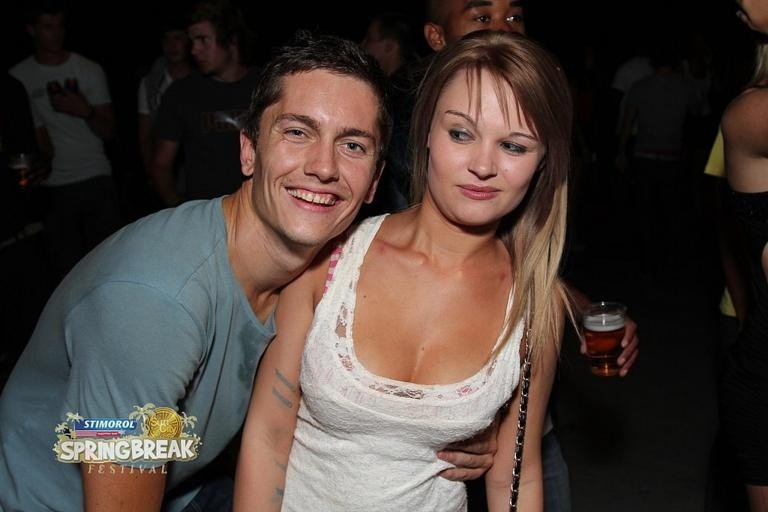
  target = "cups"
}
[9,151,33,189]
[580,301,629,379]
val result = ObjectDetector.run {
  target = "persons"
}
[0,0,768,512]
